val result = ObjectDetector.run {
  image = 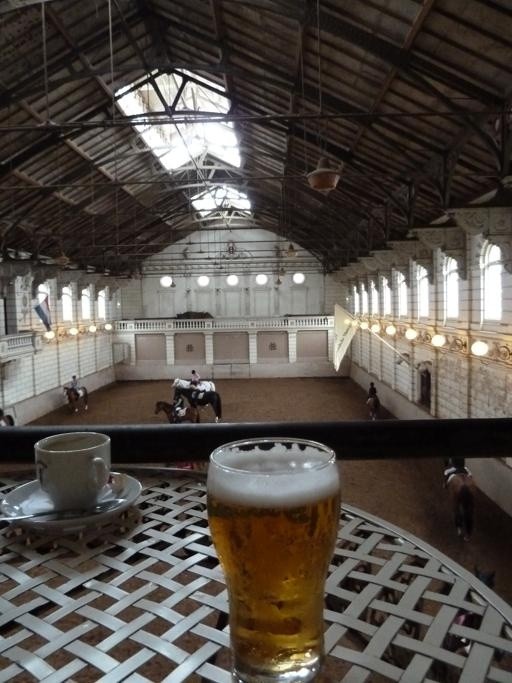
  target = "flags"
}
[332,304,359,372]
[33,297,52,333]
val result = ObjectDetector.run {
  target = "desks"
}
[0,458,512,683]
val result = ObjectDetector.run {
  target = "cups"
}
[204,434,343,682]
[34,428,116,507]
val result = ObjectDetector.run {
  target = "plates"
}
[2,468,142,531]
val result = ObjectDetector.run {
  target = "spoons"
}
[1,491,126,525]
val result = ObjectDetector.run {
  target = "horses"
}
[365,397,378,420]
[0,415,14,426]
[440,463,477,542]
[155,401,200,424]
[173,386,222,423]
[64,386,88,417]
[171,378,215,407]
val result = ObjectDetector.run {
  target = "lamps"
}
[287,215,297,257]
[305,121,340,192]
[44,324,113,342]
[343,318,496,360]
[57,242,70,267]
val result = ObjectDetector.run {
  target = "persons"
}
[71,375,80,396]
[440,456,465,489]
[189,369,201,387]
[172,391,191,417]
[368,381,377,397]
